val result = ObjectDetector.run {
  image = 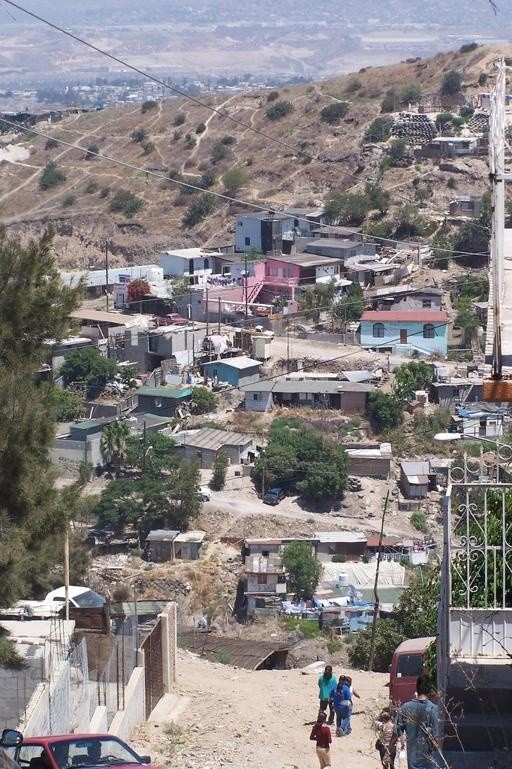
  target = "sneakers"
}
[336,725,352,736]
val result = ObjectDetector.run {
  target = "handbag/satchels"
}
[376,732,384,750]
[340,700,351,706]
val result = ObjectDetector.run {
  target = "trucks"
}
[159,313,190,326]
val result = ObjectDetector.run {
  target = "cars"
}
[0,733,163,769]
[264,488,286,506]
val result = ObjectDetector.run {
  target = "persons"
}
[329,676,360,733]
[329,674,352,737]
[381,673,447,769]
[316,665,338,725]
[86,740,110,762]
[375,712,397,769]
[310,714,332,769]
